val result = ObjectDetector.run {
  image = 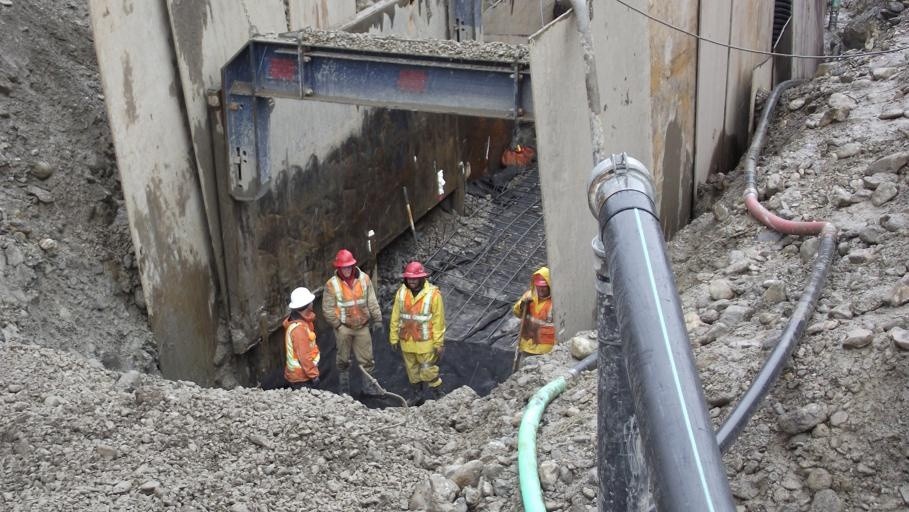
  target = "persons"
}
[321,249,387,397]
[513,267,556,369]
[389,262,446,407]
[282,287,320,391]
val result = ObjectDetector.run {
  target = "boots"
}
[360,368,388,399]
[400,379,425,407]
[337,371,352,396]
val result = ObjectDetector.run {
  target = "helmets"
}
[533,273,548,288]
[400,261,428,279]
[331,248,357,269]
[288,286,316,310]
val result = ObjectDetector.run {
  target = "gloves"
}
[313,377,321,390]
[372,320,385,337]
[390,342,399,353]
[338,325,355,343]
